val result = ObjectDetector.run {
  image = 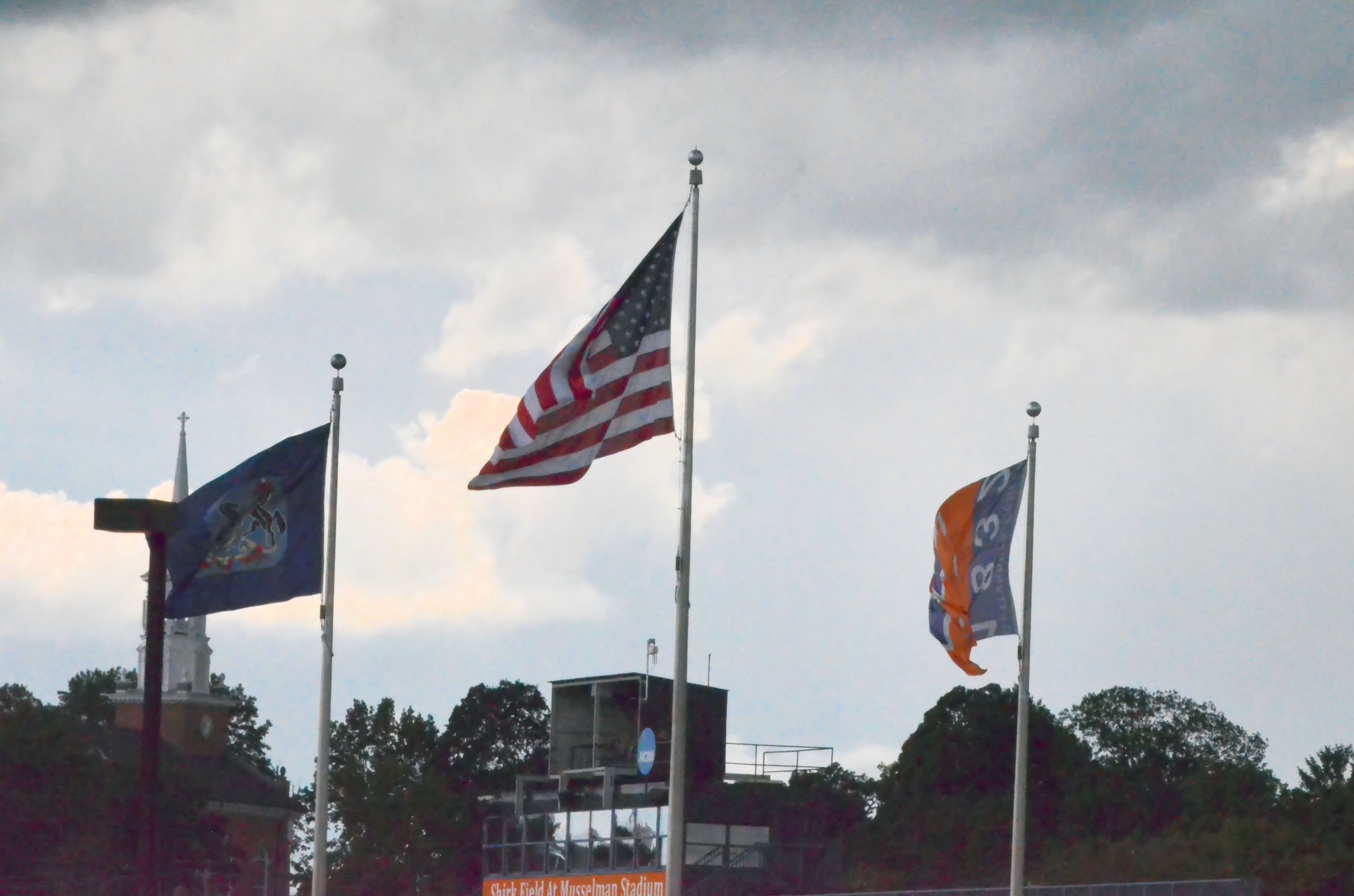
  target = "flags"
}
[162,422,334,621]
[927,457,1029,677]
[468,208,688,493]
